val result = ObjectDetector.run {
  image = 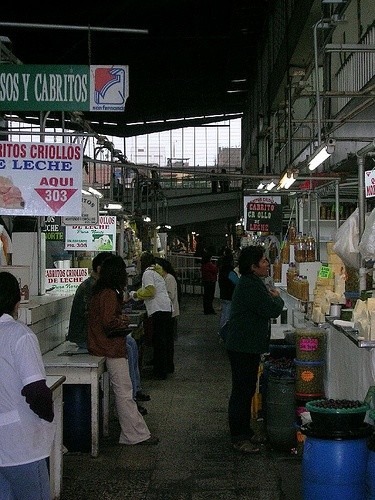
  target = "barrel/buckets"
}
[299,422,374,500]
[263,361,326,450]
[296,327,327,362]
[293,358,326,394]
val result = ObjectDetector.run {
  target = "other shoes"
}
[138,405,148,416]
[250,433,263,443]
[231,440,260,454]
[134,434,160,446]
[135,390,151,402]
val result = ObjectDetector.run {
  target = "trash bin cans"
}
[368,427,374,499]
[298,423,368,500]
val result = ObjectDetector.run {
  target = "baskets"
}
[120,309,146,324]
[305,399,368,441]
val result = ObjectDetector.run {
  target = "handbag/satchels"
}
[102,318,132,339]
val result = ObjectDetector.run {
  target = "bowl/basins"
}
[306,399,370,429]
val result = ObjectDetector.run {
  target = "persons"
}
[0,271,56,500]
[64,250,180,445]
[209,168,232,194]
[0,217,14,265]
[200,247,238,325]
[222,246,285,453]
[151,164,160,202]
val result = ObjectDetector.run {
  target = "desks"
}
[42,340,110,500]
[328,316,375,401]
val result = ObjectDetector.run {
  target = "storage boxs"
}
[295,326,327,407]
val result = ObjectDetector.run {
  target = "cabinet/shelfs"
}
[279,286,314,325]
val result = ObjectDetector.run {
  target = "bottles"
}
[292,232,315,262]
[273,256,281,283]
[286,262,309,301]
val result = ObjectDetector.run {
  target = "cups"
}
[330,305,342,317]
